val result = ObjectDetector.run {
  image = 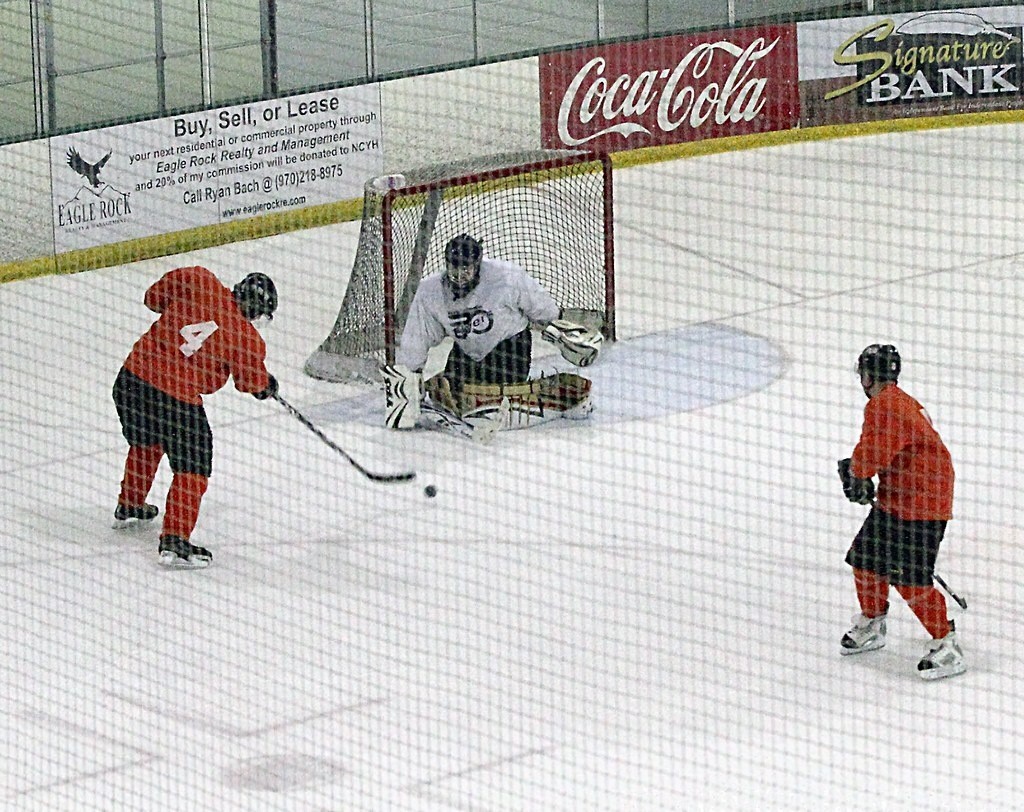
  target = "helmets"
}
[446,233,483,299]
[234,272,278,322]
[858,344,901,375]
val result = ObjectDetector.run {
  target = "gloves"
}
[837,459,875,505]
[252,373,279,400]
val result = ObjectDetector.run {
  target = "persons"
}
[839,345,968,679]
[110,266,278,570]
[380,233,598,446]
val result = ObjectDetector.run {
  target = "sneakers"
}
[110,502,159,530]
[839,601,889,655]
[158,534,212,569]
[917,620,966,680]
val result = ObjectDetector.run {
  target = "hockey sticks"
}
[420,396,510,446]
[271,394,417,483]
[869,498,968,609]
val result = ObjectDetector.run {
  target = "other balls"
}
[424,486,438,498]
